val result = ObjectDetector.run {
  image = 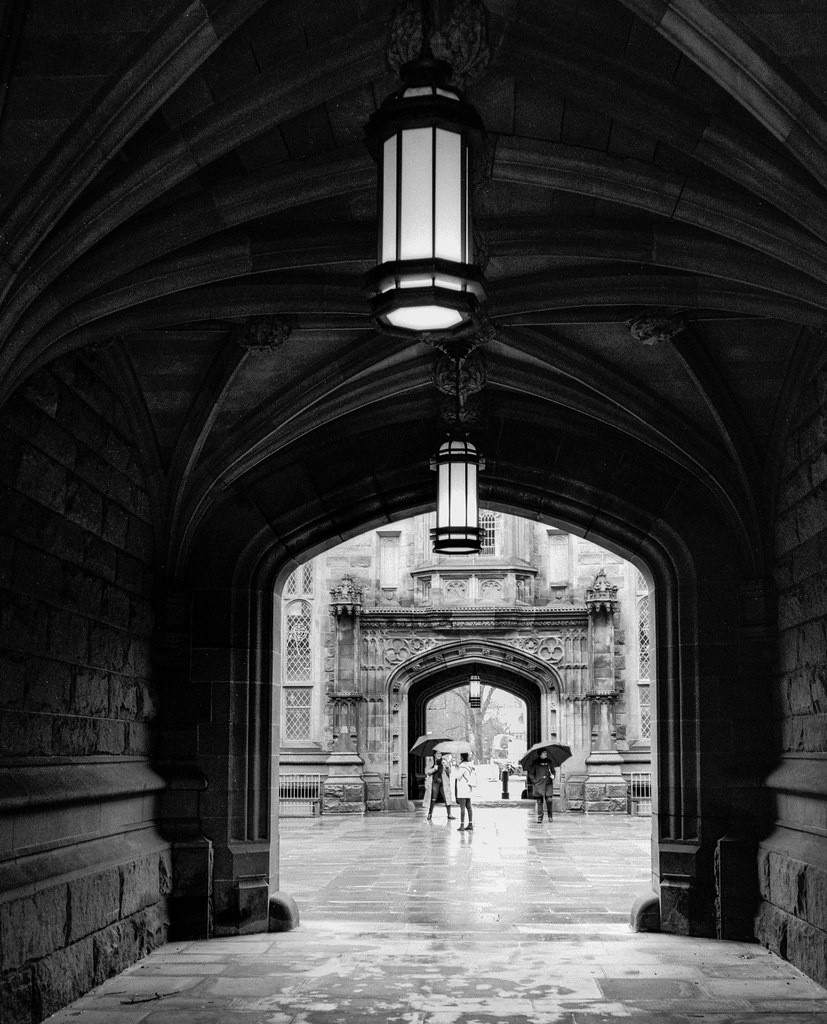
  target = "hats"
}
[537,748,547,756]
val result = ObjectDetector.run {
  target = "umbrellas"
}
[433,741,470,754]
[409,733,454,756]
[518,741,572,775]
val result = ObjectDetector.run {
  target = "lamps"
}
[429,428,485,554]
[359,0,491,346]
[469,669,480,708]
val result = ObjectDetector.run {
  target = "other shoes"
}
[465,823,473,830]
[549,817,553,822]
[537,819,542,823]
[448,814,456,819]
[457,823,465,831]
[427,813,432,820]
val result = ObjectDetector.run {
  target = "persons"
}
[425,748,555,830]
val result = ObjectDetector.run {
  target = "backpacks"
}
[462,762,477,788]
